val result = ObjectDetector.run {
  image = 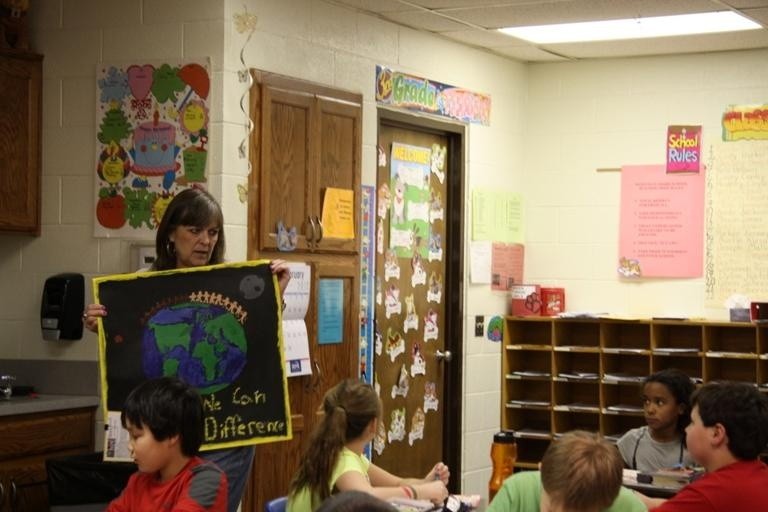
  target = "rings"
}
[82,312,88,320]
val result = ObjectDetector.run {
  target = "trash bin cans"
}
[45,451,139,512]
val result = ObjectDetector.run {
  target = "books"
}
[605,402,646,413]
[652,346,700,353]
[512,426,550,437]
[601,370,648,382]
[601,432,623,440]
[556,371,598,381]
[508,398,551,407]
[553,400,600,411]
[511,369,550,377]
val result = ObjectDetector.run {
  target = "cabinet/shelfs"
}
[252,254,361,511]
[0,46,44,240]
[0,403,103,512]
[500,313,767,477]
[257,84,361,254]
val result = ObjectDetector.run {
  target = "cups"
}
[1,374,15,399]
[511,282,542,317]
[541,287,565,314]
[750,302,768,323]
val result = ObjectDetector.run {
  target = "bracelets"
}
[400,484,418,500]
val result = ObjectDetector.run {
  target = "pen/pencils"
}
[434,468,441,481]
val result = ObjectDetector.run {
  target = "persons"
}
[483,427,652,511]
[285,375,450,512]
[101,374,230,512]
[80,186,293,510]
[643,378,768,510]
[613,367,707,470]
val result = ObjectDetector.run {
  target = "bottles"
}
[488,430,520,490]
